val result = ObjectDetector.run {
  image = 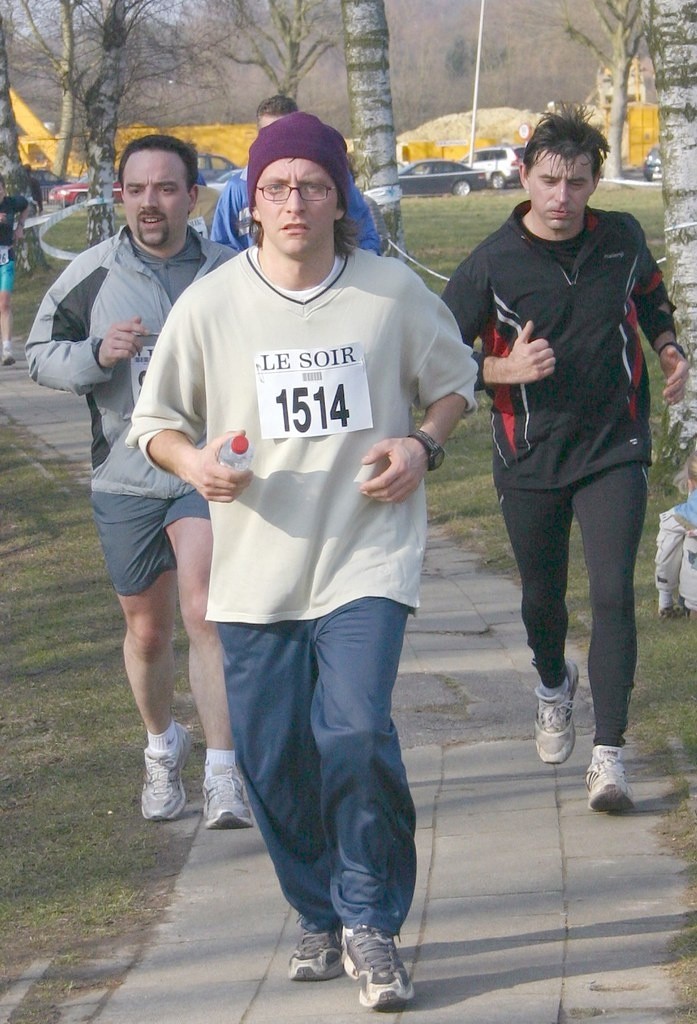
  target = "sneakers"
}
[141,722,190,820]
[199,764,253,828]
[585,745,636,811]
[341,922,415,1008]
[532,655,580,764]
[288,924,342,980]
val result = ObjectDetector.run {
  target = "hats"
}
[248,109,350,213]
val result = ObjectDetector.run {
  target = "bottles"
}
[217,435,254,471]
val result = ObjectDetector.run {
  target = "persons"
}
[0,177,28,366]
[210,95,380,254]
[441,101,686,807]
[24,164,43,215]
[125,112,479,1012]
[655,449,697,622]
[26,135,249,830]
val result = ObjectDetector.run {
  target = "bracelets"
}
[658,341,684,357]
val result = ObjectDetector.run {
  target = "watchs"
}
[409,430,445,471]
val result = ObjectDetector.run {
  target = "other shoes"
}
[1,352,16,366]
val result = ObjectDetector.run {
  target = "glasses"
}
[255,182,336,202]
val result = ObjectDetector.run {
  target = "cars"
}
[197,154,241,182]
[398,160,487,197]
[30,170,71,198]
[206,167,245,192]
[642,145,664,181]
[48,170,123,209]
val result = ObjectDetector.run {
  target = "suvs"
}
[461,147,527,190]
